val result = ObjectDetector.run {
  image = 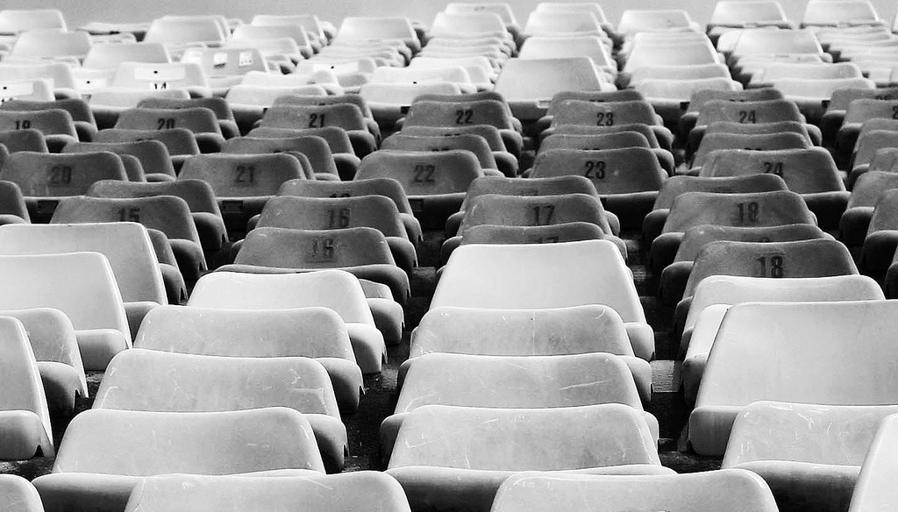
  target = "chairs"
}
[0,0,896,512]
